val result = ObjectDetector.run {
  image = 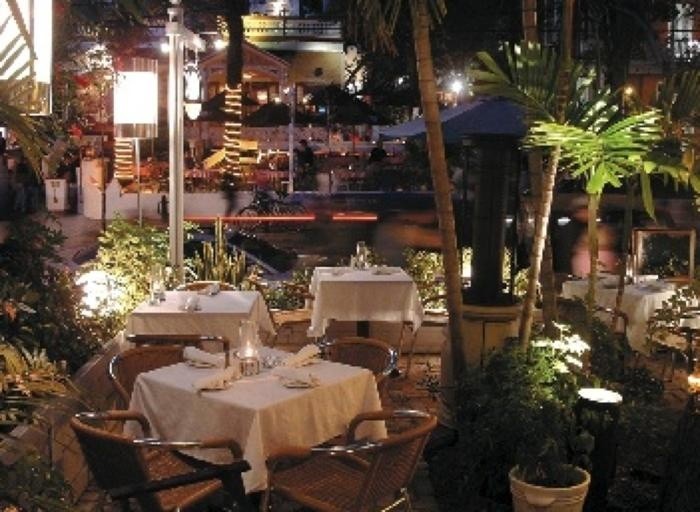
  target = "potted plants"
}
[455,335,590,510]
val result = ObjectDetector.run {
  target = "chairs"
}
[322,338,396,392]
[175,279,240,292]
[69,407,253,511]
[257,408,438,512]
[108,344,188,407]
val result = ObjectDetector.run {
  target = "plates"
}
[189,349,315,390]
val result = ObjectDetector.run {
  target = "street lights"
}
[165,5,208,290]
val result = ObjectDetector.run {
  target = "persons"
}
[333,127,345,140]
[364,127,378,142]
[295,139,313,169]
[348,130,358,143]
[367,139,388,164]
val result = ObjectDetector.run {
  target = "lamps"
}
[0,0,70,117]
[113,71,158,227]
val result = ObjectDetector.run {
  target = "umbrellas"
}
[243,102,309,131]
[193,92,259,124]
[305,98,396,154]
[303,83,356,129]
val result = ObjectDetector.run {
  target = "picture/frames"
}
[631,227,696,282]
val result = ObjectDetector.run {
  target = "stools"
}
[576,386,624,475]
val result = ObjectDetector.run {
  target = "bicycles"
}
[234,182,311,234]
